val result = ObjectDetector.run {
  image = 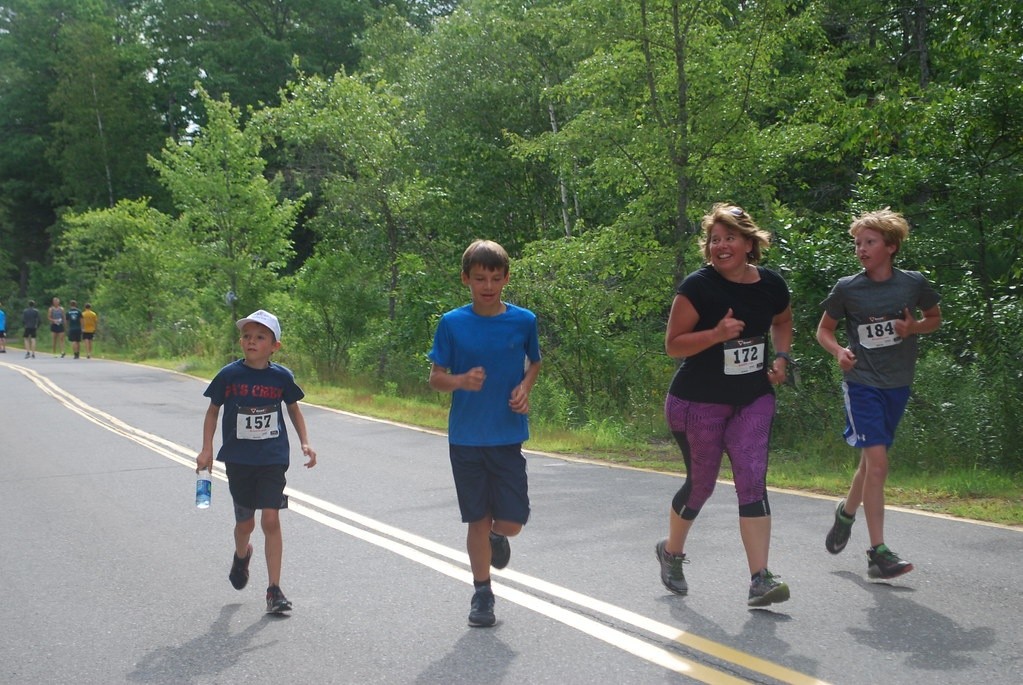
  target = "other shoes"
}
[0,349,89,360]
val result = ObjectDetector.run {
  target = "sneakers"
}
[655,540,690,595]
[825,500,855,553]
[748,568,790,606]
[488,529,511,570]
[266,587,292,613]
[867,544,913,579]
[468,585,497,627]
[229,543,253,590]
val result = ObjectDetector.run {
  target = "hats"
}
[236,309,281,341]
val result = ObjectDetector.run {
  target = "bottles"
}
[195,466,212,509]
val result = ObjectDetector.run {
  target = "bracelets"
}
[774,351,798,367]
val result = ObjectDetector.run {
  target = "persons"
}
[48,297,67,358]
[22,300,42,358]
[429,240,541,627]
[81,304,98,359]
[815,207,942,579]
[65,300,85,360]
[195,309,319,614]
[0,303,7,353]
[654,204,799,607]
